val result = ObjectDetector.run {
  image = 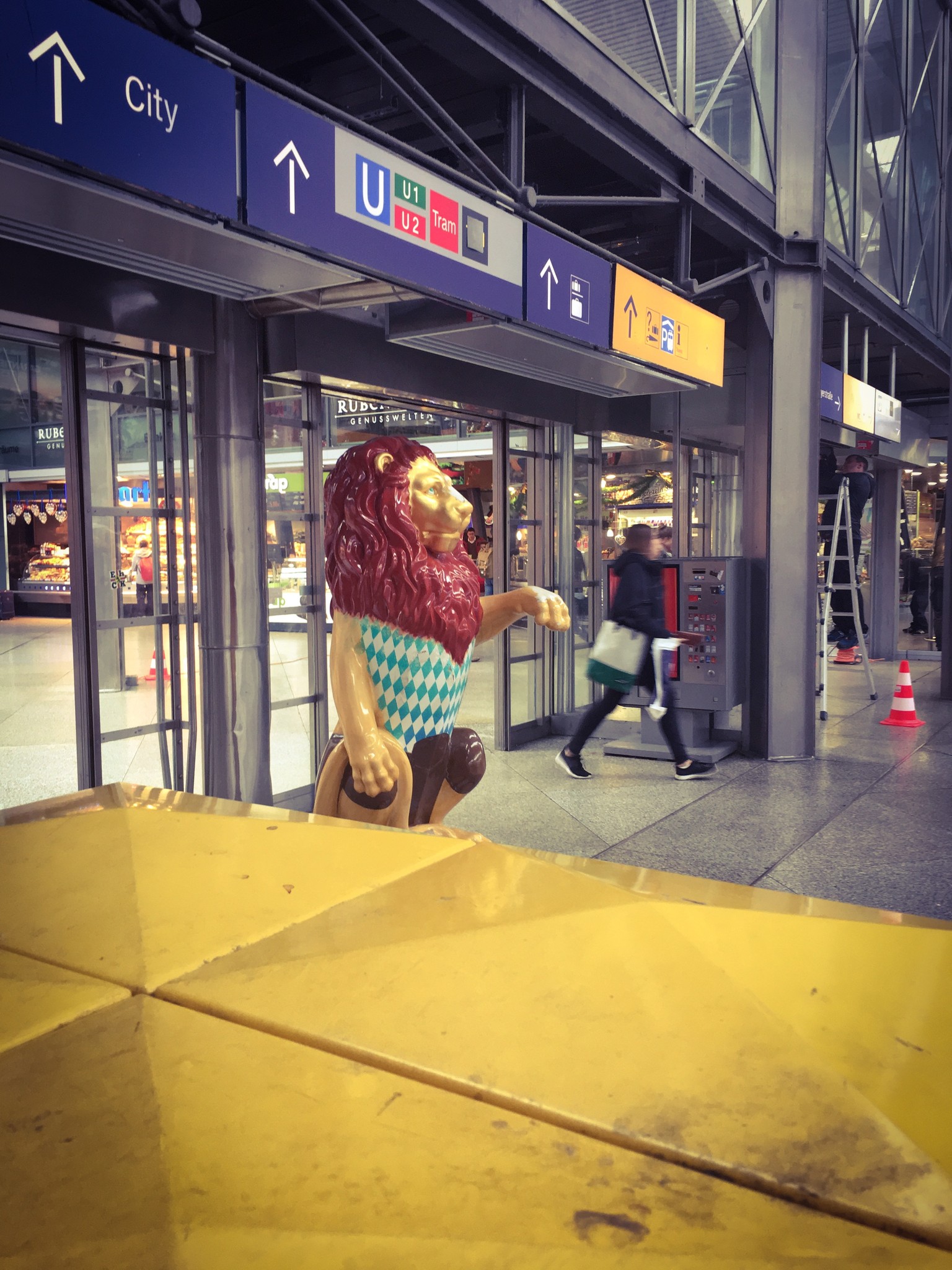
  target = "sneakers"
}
[827,626,847,642]
[675,758,719,780]
[555,749,592,779]
[836,628,868,649]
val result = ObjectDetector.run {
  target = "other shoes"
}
[902,622,917,633]
[912,627,929,635]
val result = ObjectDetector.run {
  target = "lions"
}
[312,437,572,831]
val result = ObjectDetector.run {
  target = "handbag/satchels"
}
[587,619,644,676]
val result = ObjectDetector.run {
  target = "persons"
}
[573,525,588,642]
[900,551,932,635]
[818,454,874,648]
[555,524,719,781]
[929,532,946,652]
[132,539,154,617]
[484,527,527,596]
[463,527,486,564]
[654,522,673,559]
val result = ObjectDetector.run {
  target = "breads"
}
[119,518,196,579]
[29,543,70,582]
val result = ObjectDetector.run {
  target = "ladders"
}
[814,476,879,721]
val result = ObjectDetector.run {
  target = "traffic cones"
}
[145,650,157,681]
[162,651,170,680]
[880,660,926,727]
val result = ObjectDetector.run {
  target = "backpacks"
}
[137,556,153,582]
